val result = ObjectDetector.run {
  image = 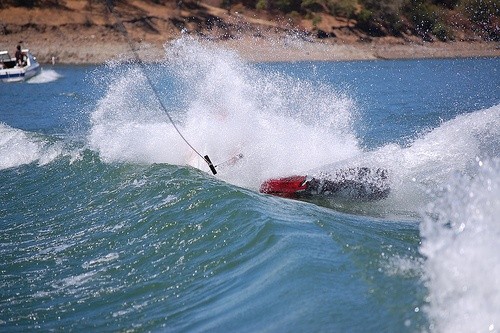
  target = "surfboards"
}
[260,167,391,202]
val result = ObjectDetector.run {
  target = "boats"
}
[0,49,42,84]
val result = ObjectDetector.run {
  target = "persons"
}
[14,45,25,66]
